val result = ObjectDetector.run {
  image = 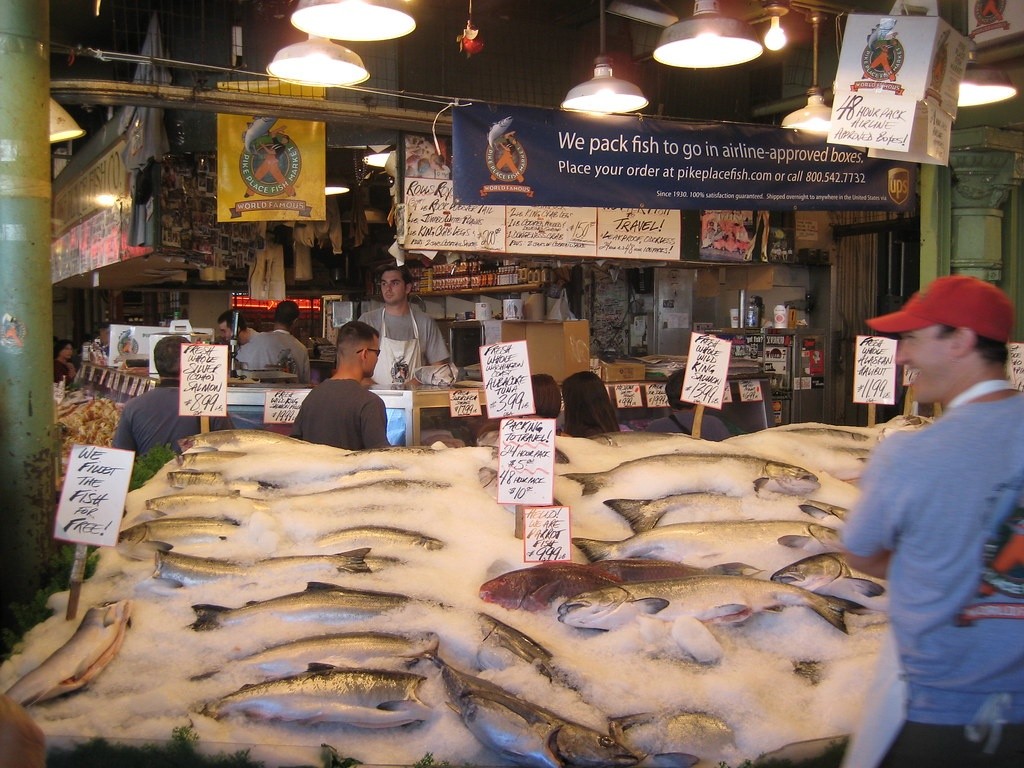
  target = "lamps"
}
[561,1,648,114]
[954,0,1017,106]
[782,11,834,133]
[50,97,86,144]
[653,1,764,69]
[291,0,418,40]
[265,34,370,88]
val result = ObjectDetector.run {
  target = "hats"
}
[864,275,1012,344]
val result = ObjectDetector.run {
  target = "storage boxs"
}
[827,0,1024,167]
[500,318,591,385]
[599,359,646,383]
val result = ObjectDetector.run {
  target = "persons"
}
[235,300,310,384]
[703,210,750,254]
[217,309,259,344]
[496,374,561,436]
[556,371,634,438]
[841,275,1024,768]
[359,261,451,384]
[289,321,390,450]
[111,336,234,457]
[98,323,111,356]
[644,368,730,443]
[770,244,792,260]
[53,336,77,386]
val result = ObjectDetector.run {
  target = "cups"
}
[730,308,739,328]
[503,299,523,320]
[475,303,492,321]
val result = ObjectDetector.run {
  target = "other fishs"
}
[4,598,130,712]
[118,516,452,730]
[476,417,922,598]
[476,611,579,696]
[145,433,454,523]
[477,560,868,636]
[430,653,733,768]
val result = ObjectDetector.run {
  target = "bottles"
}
[411,270,419,292]
[82,339,92,361]
[174,311,179,319]
[774,304,788,329]
[102,345,108,365]
[745,298,759,327]
[801,347,806,358]
[433,258,554,291]
[159,315,173,327]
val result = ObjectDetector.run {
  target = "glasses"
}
[356,346,381,357]
